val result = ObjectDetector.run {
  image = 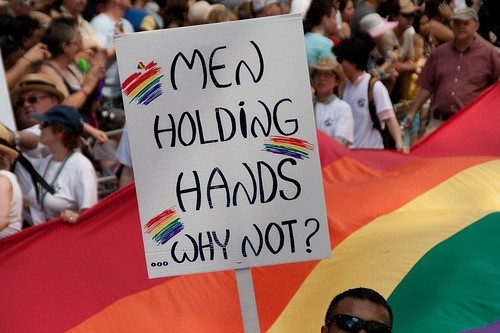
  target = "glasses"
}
[311,71,335,78]
[326,313,392,333]
[67,36,84,46]
[40,121,55,129]
[15,94,53,106]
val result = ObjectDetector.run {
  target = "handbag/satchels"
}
[382,127,396,149]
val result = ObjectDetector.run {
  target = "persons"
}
[1,0,500,237]
[320,287,394,333]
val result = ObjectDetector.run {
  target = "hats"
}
[31,105,84,136]
[12,74,64,103]
[451,7,478,21]
[253,0,279,10]
[188,1,227,20]
[361,13,398,37]
[400,0,419,13]
[0,124,18,158]
[309,58,345,79]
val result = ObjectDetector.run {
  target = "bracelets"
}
[79,88,88,98]
[20,53,32,65]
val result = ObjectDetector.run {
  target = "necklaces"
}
[38,150,75,205]
